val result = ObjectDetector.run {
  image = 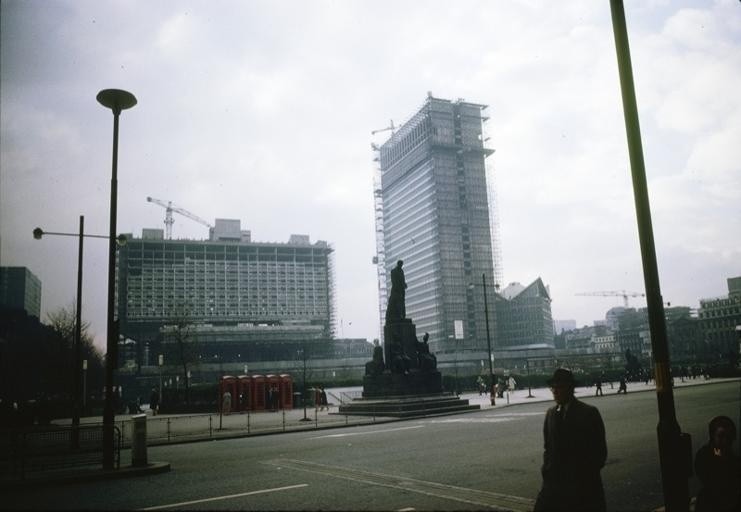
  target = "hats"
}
[546,369,581,387]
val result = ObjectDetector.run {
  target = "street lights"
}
[467,274,504,405]
[92,85,139,483]
[82,358,88,417]
[31,215,128,451]
[157,353,164,413]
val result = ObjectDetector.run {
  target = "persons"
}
[321,388,329,411]
[366,338,384,375]
[386,260,408,321]
[222,388,233,415]
[596,366,701,397]
[695,415,741,511]
[477,373,517,398]
[314,386,321,411]
[419,331,438,373]
[147,387,159,416]
[534,368,608,510]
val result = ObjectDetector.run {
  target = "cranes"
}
[144,196,214,241]
[371,118,401,137]
[573,287,644,308]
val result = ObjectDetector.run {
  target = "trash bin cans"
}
[294,392,302,409]
